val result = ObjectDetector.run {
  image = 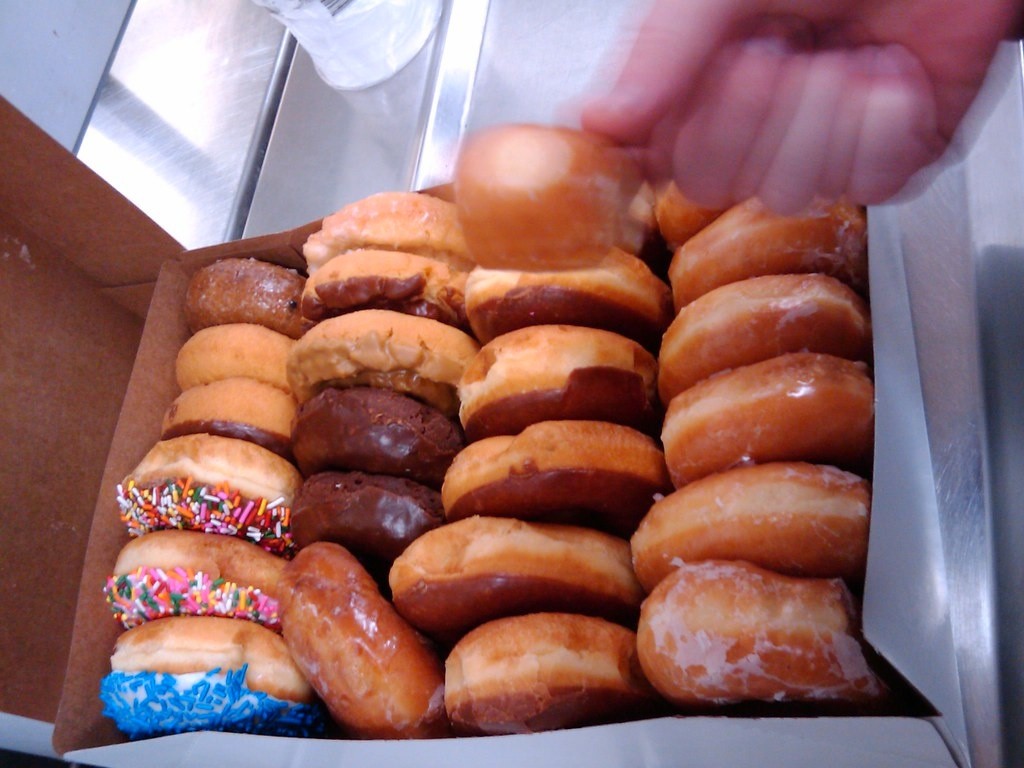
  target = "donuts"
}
[91,124,889,735]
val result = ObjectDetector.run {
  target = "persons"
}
[581,0,1024,216]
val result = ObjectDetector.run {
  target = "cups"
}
[254,0,442,89]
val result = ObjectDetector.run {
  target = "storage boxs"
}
[0,90,970,768]
